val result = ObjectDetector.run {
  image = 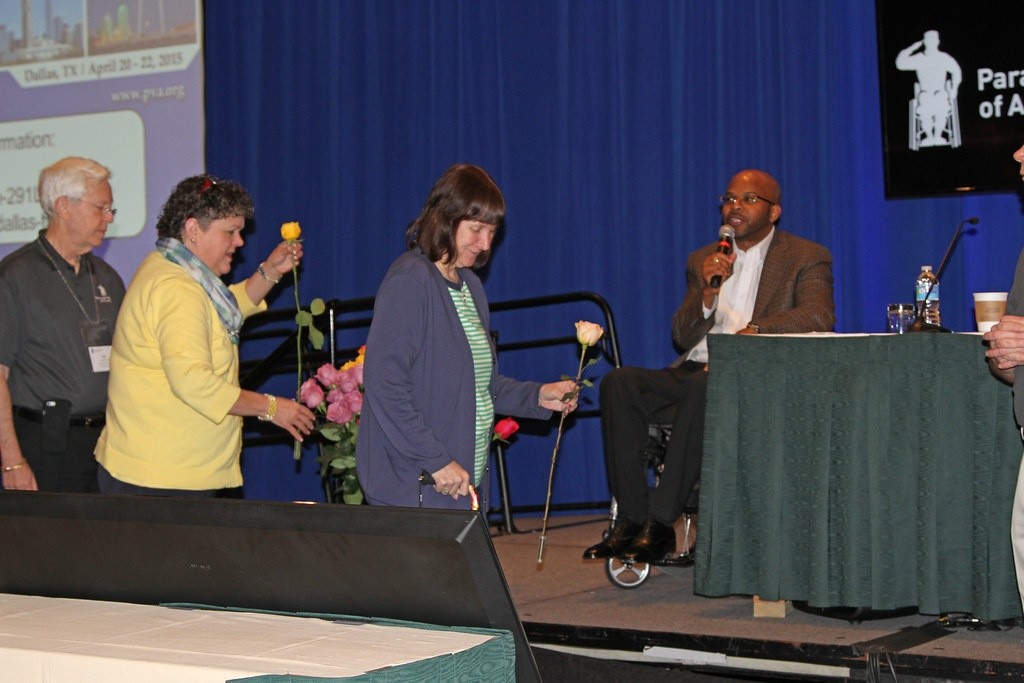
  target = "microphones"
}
[909,217,980,332]
[710,224,735,289]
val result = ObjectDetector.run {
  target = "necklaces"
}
[446,269,467,305]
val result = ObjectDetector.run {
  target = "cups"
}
[972,291,1009,331]
[887,303,915,334]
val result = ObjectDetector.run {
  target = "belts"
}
[11,404,105,428]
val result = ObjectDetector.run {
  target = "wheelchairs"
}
[600,422,697,589]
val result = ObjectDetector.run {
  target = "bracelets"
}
[1,459,26,472]
[257,393,277,421]
[257,261,283,283]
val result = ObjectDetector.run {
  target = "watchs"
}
[746,321,759,334]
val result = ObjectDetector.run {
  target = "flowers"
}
[296,346,366,505]
[281,222,326,461]
[490,416,519,445]
[539,319,603,567]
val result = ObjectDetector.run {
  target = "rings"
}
[442,488,449,494]
[711,257,720,265]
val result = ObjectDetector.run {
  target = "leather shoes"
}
[620,524,677,562]
[582,511,648,560]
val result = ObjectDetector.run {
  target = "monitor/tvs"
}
[875,0,1024,200]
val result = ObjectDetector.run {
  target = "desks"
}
[0,593,516,683]
[694,334,1024,618]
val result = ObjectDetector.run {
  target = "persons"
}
[355,164,579,510]
[0,156,126,491]
[981,144,1024,615]
[580,168,836,561]
[94,173,316,498]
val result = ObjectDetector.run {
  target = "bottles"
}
[916,266,940,326]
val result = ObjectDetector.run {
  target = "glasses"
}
[65,196,116,216]
[719,191,774,207]
[183,175,221,222]
